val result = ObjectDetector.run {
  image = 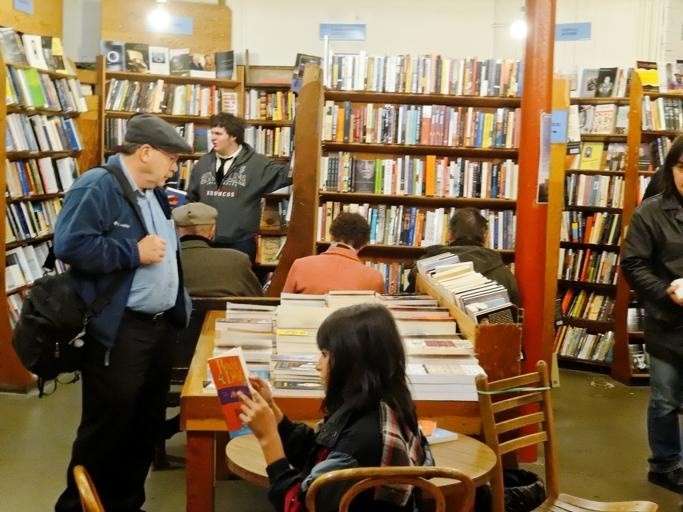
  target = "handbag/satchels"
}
[11,164,150,399]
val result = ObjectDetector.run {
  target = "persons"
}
[619,135,683,494]
[641,166,667,201]
[404,207,519,317]
[184,112,294,271]
[236,304,435,512]
[280,212,386,293]
[12,113,195,512]
[170,202,265,297]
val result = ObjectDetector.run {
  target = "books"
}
[629,343,651,374]
[244,88,297,120]
[0,27,75,75]
[568,105,580,143]
[670,278,683,301]
[319,151,519,200]
[321,99,521,149]
[327,52,523,99]
[315,201,516,250]
[244,125,294,158]
[105,78,239,118]
[627,306,647,332]
[639,136,672,171]
[99,40,237,82]
[203,290,488,402]
[6,113,85,154]
[5,240,71,294]
[208,346,259,439]
[416,251,527,362]
[255,235,287,267]
[633,59,683,93]
[6,156,79,199]
[566,174,625,209]
[553,325,615,361]
[8,288,31,330]
[366,261,413,295]
[291,53,323,92]
[558,247,620,285]
[165,187,187,211]
[641,95,683,132]
[167,160,200,192]
[172,122,214,154]
[5,65,90,115]
[105,118,128,153]
[594,67,630,97]
[277,197,292,230]
[6,197,62,244]
[417,419,458,446]
[566,142,626,171]
[560,287,616,321]
[561,211,622,246]
[639,175,651,203]
[576,104,630,135]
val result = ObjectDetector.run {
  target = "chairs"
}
[305,466,475,512]
[474,359,659,512]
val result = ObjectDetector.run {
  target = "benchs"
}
[155,296,282,471]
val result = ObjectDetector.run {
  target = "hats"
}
[125,113,193,154]
[171,201,219,225]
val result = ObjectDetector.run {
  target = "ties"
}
[216,157,234,189]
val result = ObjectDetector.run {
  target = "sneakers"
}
[646,467,683,495]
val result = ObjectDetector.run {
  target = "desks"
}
[181,308,481,511]
[224,434,497,501]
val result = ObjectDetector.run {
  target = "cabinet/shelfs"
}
[314,86,523,269]
[611,71,683,387]
[417,270,523,470]
[555,96,632,375]
[98,55,295,291]
[0,32,101,395]
